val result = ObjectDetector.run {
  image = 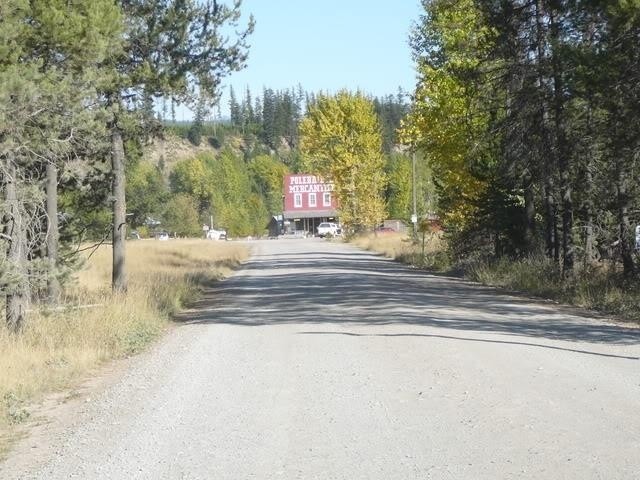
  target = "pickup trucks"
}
[316,222,338,238]
